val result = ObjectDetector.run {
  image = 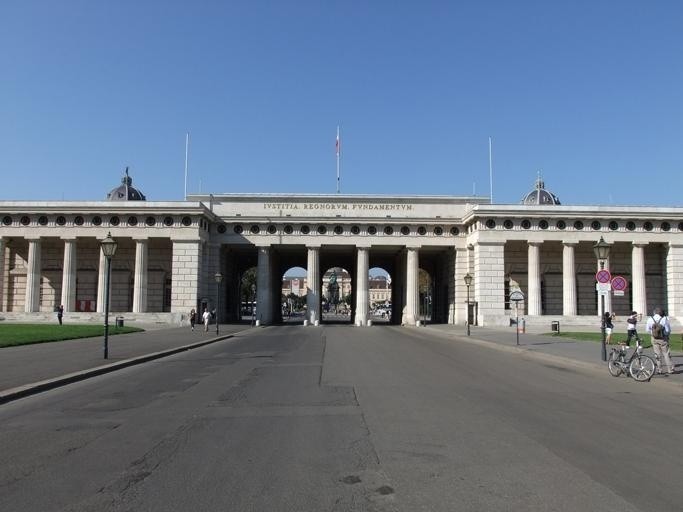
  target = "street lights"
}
[464,272,473,337]
[100,230,119,359]
[213,270,224,336]
[592,235,613,362]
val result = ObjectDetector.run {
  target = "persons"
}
[189,308,196,331]
[625,311,643,349]
[56,305,62,325]
[646,306,681,375]
[603,312,615,344]
[201,307,212,332]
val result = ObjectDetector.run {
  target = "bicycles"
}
[608,336,656,382]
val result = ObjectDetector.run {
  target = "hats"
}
[604,312,610,316]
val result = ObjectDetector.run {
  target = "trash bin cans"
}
[116,317,123,327]
[552,321,559,334]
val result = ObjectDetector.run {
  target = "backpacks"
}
[651,316,666,339]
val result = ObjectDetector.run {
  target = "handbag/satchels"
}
[609,324,614,329]
[627,318,637,324]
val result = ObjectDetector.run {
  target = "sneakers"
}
[626,345,630,349]
[669,370,675,374]
[638,345,643,348]
[656,370,662,374]
